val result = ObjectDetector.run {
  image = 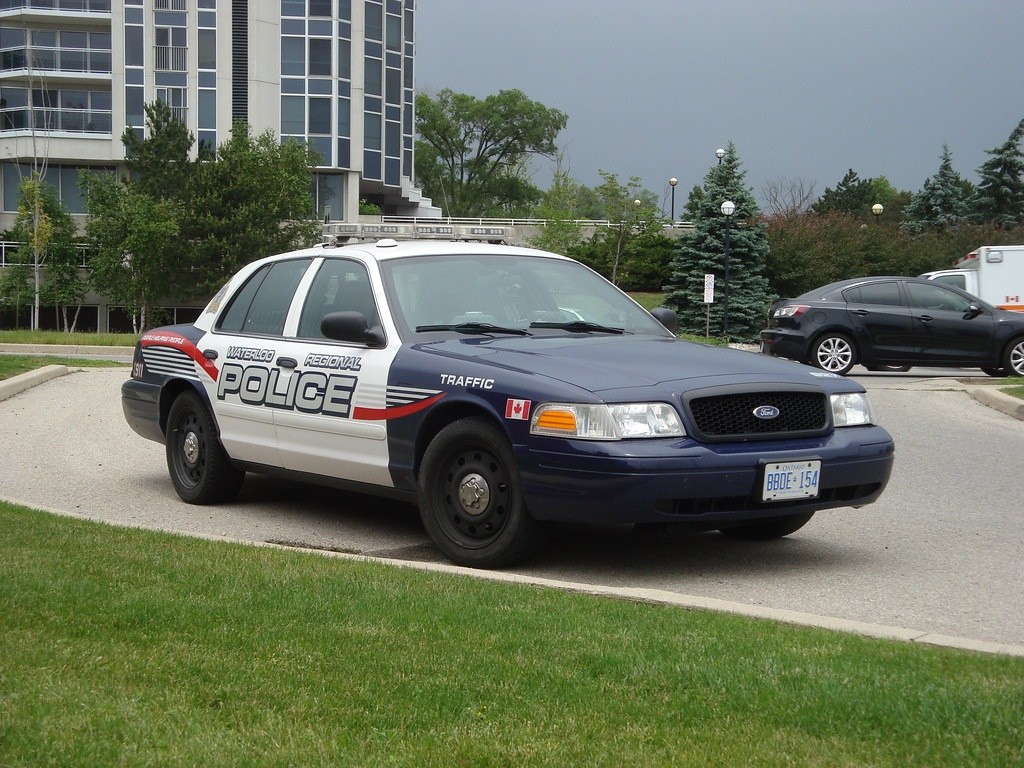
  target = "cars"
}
[758,276,1024,377]
[121,239,895,569]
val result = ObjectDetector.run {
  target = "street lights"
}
[634,200,641,220]
[669,177,678,220]
[721,201,735,331]
[715,149,725,165]
[871,203,883,224]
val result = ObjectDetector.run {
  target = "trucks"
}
[916,245,1024,312]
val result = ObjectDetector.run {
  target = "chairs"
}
[331,280,377,331]
